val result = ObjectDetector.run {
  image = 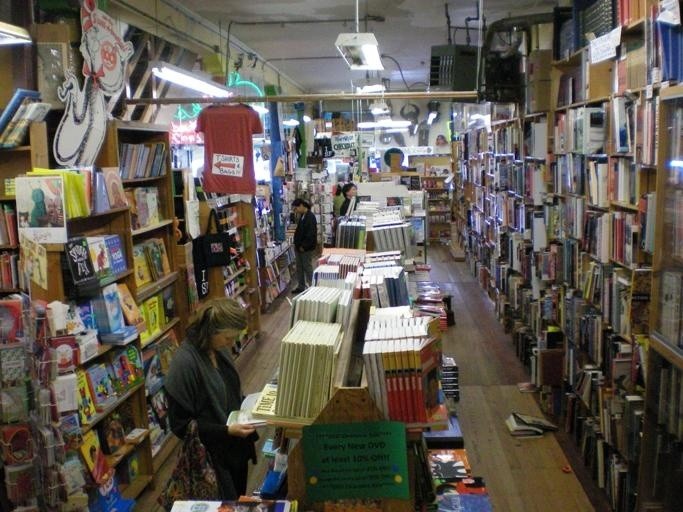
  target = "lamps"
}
[147,2,240,100]
[332,1,415,115]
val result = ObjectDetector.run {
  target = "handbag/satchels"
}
[192,208,230,270]
[156,419,224,512]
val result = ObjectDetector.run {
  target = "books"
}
[169,175,490,511]
[410,1,679,510]
[0,19,334,509]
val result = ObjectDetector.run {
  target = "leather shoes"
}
[291,289,300,294]
